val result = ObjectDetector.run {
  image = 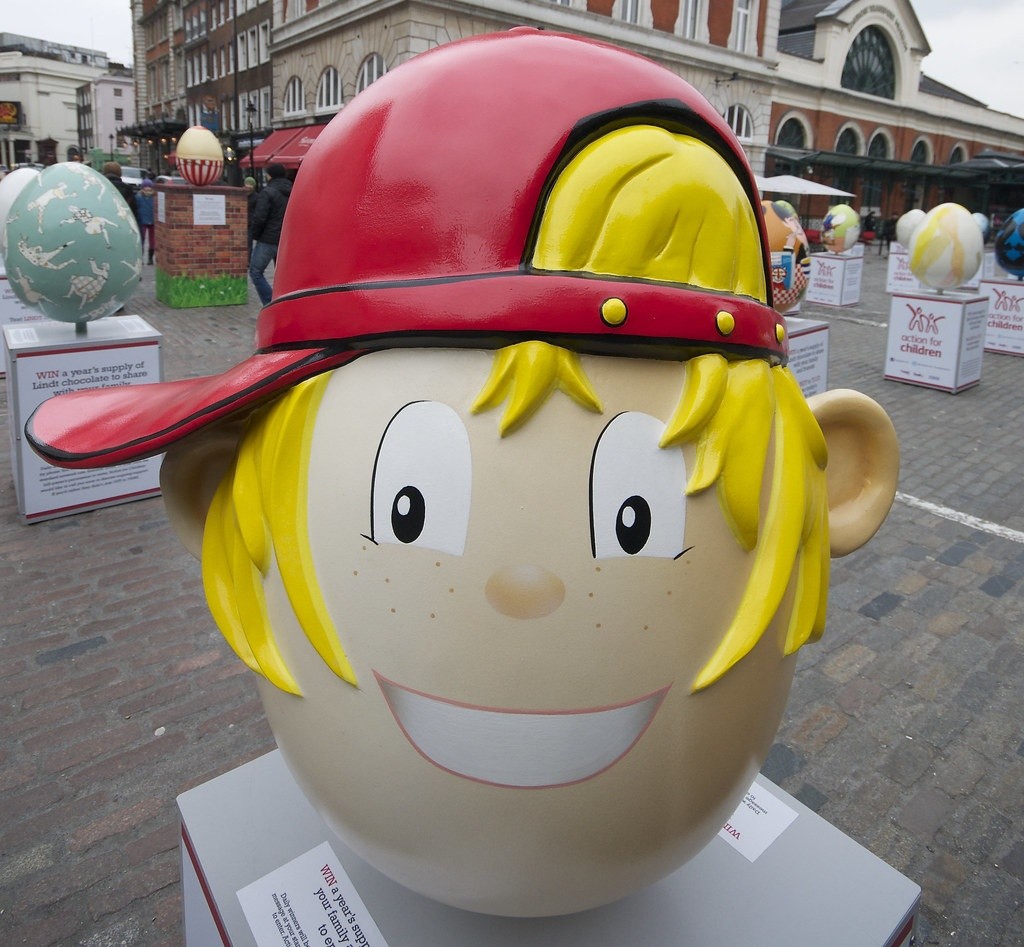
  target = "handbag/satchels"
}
[863,231,875,240]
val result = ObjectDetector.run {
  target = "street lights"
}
[243,102,259,177]
[107,134,114,162]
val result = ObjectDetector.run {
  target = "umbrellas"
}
[752,175,857,229]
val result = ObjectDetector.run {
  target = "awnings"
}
[239,125,328,169]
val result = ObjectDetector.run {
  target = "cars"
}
[155,173,188,185]
[119,166,151,195]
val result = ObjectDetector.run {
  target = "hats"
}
[244,177,257,186]
[102,161,122,177]
[891,209,898,215]
[26,29,792,468]
[142,178,152,186]
[265,163,285,178]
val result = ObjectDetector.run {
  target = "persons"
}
[249,162,293,306]
[885,212,898,258]
[244,177,261,267]
[135,178,154,264]
[103,162,138,221]
[866,212,876,247]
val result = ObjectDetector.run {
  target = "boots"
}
[147,248,154,264]
